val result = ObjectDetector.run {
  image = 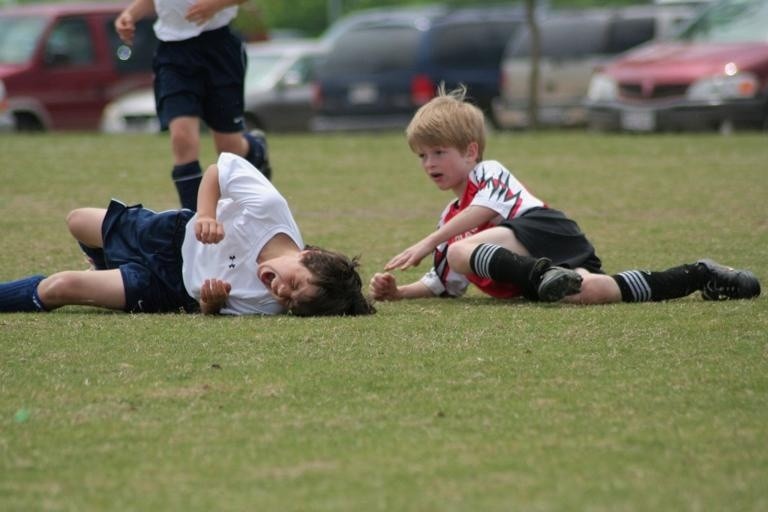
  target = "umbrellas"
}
[248,129,271,181]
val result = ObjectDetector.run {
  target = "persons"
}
[1,151,373,315]
[370,80,761,304]
[114,1,271,209]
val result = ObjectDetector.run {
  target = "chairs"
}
[696,258,761,300]
[528,257,583,302]
